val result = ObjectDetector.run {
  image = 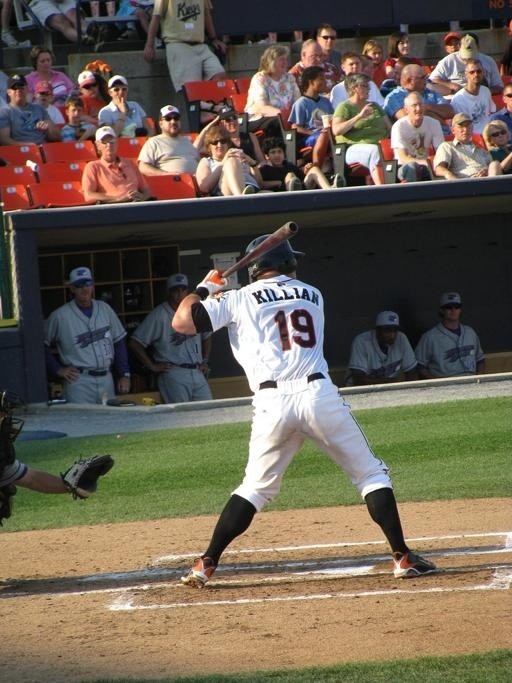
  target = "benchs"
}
[110,350,512,406]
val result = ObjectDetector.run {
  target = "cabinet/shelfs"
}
[37,243,182,399]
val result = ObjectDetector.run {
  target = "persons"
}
[40,266,133,405]
[83,0,116,17]
[412,290,486,379]
[127,272,216,404]
[144,0,228,95]
[342,308,418,386]
[116,0,163,48]
[0,20,511,204]
[0,406,114,528]
[169,232,445,590]
[1,0,20,46]
[21,0,108,51]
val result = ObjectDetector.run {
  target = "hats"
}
[218,107,235,120]
[168,273,188,289]
[7,74,53,92]
[440,292,460,306]
[160,105,180,117]
[376,311,399,328]
[77,71,128,88]
[70,267,93,286]
[444,32,477,60]
[95,126,116,141]
[452,113,472,127]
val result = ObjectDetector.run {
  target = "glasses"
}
[324,36,335,40]
[110,88,126,92]
[490,130,507,137]
[504,94,512,97]
[162,117,179,120]
[210,139,226,145]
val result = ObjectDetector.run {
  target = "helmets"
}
[246,234,304,278]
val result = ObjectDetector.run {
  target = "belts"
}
[79,366,108,375]
[260,372,324,389]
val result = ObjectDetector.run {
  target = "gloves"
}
[196,269,227,301]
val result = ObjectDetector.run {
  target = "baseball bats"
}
[221,221,299,278]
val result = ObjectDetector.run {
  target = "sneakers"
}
[392,552,436,579]
[180,557,215,588]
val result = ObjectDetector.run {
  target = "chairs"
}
[1,0,512,211]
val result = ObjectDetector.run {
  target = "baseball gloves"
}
[62,453,113,499]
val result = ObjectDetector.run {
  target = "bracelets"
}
[121,371,132,379]
[203,356,210,364]
